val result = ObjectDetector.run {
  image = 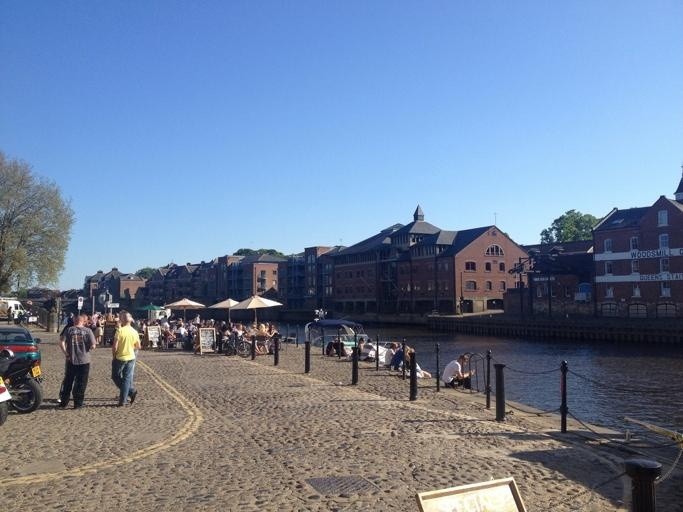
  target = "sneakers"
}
[57,399,67,410]
[120,389,138,406]
[73,406,82,410]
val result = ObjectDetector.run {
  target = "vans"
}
[0,299,26,320]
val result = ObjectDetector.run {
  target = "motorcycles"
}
[0,350,41,413]
[0,377,10,428]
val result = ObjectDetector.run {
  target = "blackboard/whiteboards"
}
[147,326,162,342]
[199,328,216,352]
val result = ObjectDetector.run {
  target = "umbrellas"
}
[138,298,206,322]
[208,295,283,329]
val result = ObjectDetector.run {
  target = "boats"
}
[302,319,403,367]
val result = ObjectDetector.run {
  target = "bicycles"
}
[222,333,252,357]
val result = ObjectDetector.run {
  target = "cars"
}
[0,326,40,371]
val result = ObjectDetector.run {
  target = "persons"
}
[326,337,476,389]
[59,311,142,409]
[7,306,12,325]
[66,313,280,354]
